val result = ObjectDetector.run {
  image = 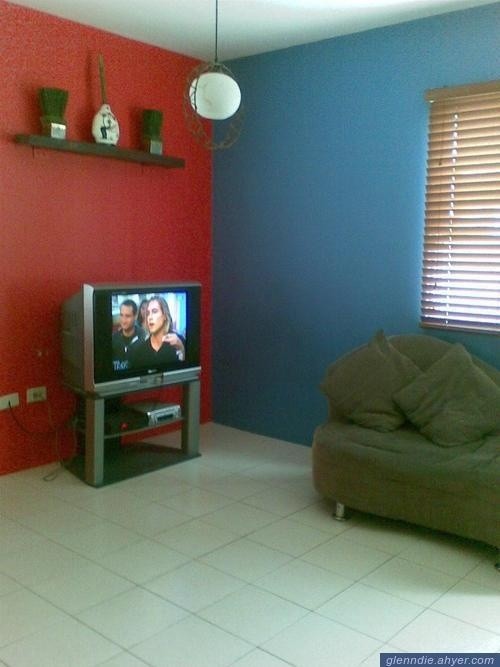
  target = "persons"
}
[112,299,146,378]
[128,295,186,372]
[137,299,149,338]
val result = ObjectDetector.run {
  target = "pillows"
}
[318,329,424,433]
[393,343,500,447]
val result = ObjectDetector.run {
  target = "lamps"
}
[181,0,247,150]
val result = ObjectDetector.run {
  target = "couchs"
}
[312,334,500,572]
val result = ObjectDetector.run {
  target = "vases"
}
[92,104,120,146]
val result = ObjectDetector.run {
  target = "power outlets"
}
[0,392,19,411]
[26,387,47,403]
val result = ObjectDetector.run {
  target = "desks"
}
[59,380,201,484]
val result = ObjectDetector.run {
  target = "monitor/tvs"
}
[59,278,203,397]
